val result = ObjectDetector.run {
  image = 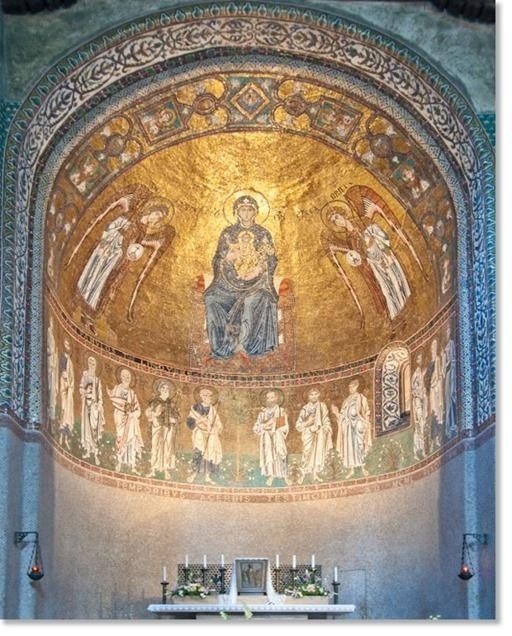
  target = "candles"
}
[333,567,339,582]
[183,554,225,568]
[275,555,316,570]
[162,568,169,582]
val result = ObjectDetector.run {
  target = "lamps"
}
[459,531,487,581]
[14,531,46,581]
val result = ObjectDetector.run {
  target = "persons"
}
[295,390,331,483]
[331,380,373,478]
[326,209,414,320]
[187,388,222,485]
[80,356,103,467]
[145,385,181,480]
[110,370,144,476]
[46,316,74,451]
[253,391,293,487]
[405,324,457,458]
[203,196,280,357]
[78,205,167,313]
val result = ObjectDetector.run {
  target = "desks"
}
[145,602,356,620]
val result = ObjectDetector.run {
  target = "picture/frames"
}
[236,559,267,594]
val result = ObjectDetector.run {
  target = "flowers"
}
[166,571,214,599]
[291,571,335,597]
